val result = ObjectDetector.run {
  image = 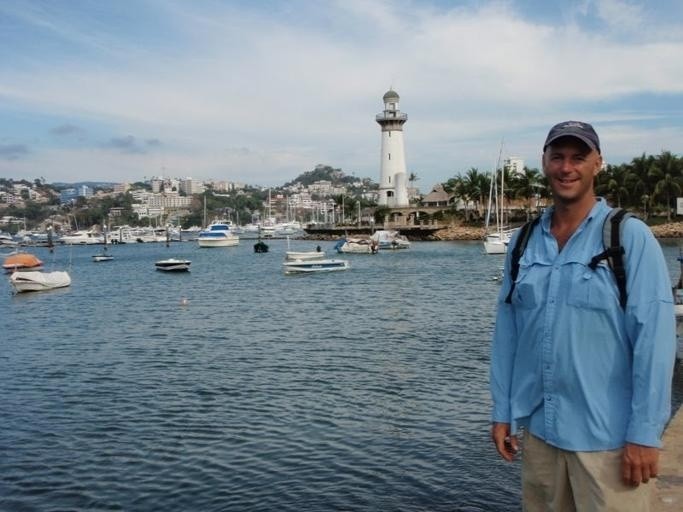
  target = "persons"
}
[489,121,677,512]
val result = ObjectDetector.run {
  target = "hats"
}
[545,121,601,152]
